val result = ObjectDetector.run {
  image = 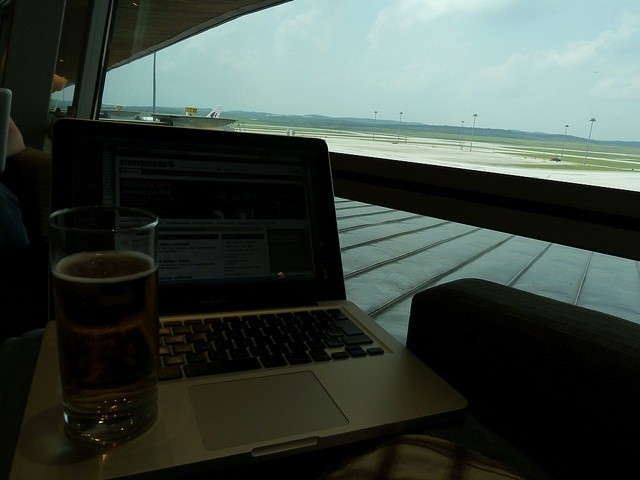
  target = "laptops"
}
[0,87,12,173]
[7,118,468,480]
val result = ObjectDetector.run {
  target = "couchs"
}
[243,276,640,478]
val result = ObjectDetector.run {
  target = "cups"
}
[47,204,160,449]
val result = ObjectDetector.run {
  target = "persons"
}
[1,2,31,253]
